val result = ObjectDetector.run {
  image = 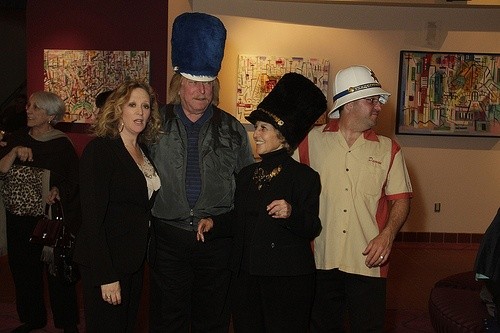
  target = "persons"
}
[0,91,79,333]
[76,78,162,333]
[196,72,328,333]
[135,72,255,333]
[292,66,414,333]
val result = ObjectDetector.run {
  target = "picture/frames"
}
[395,50,500,138]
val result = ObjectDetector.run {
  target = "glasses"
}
[365,98,384,106]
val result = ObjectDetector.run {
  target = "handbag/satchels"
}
[28,198,74,281]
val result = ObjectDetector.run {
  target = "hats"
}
[172,12,227,81]
[245,72,328,149]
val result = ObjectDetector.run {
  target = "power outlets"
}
[435,203,440,212]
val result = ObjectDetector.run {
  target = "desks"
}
[429,272,488,333]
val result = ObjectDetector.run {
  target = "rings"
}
[380,256,384,259]
[106,296,111,299]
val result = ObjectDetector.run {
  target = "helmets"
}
[328,64,390,119]
